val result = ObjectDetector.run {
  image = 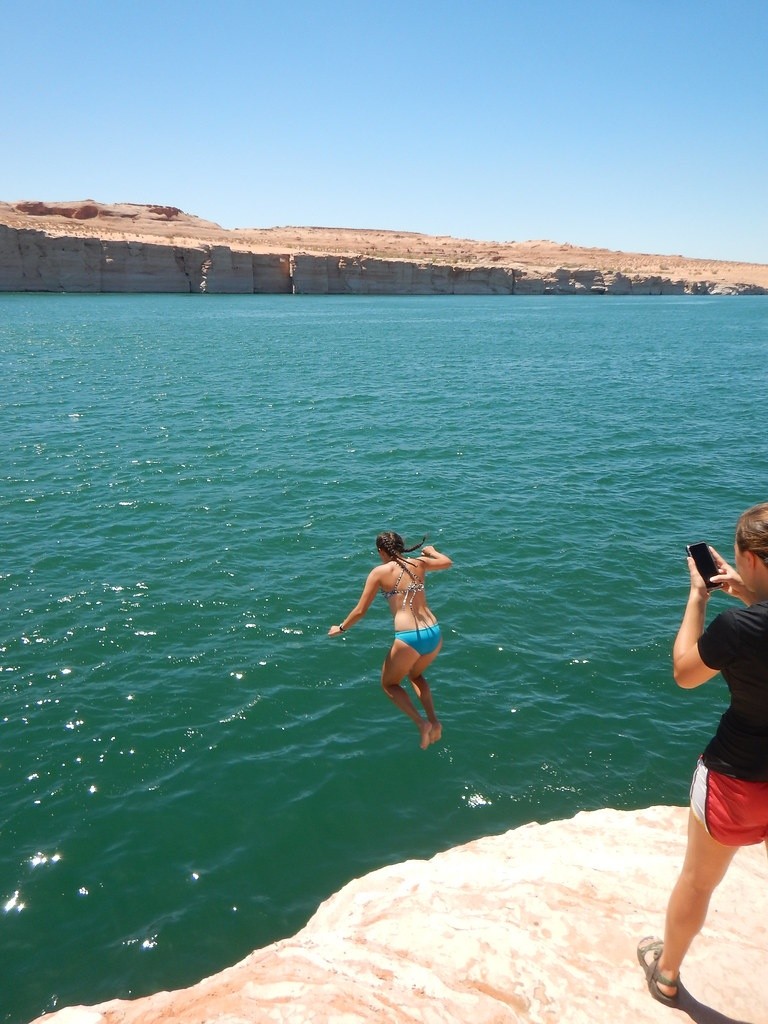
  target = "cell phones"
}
[686,542,723,590]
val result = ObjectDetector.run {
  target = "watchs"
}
[340,624,346,631]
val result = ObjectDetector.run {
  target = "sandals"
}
[637,936,681,1007]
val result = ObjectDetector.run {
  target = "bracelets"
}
[429,550,434,556]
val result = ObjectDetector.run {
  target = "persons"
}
[328,531,452,750]
[637,503,768,1006]
